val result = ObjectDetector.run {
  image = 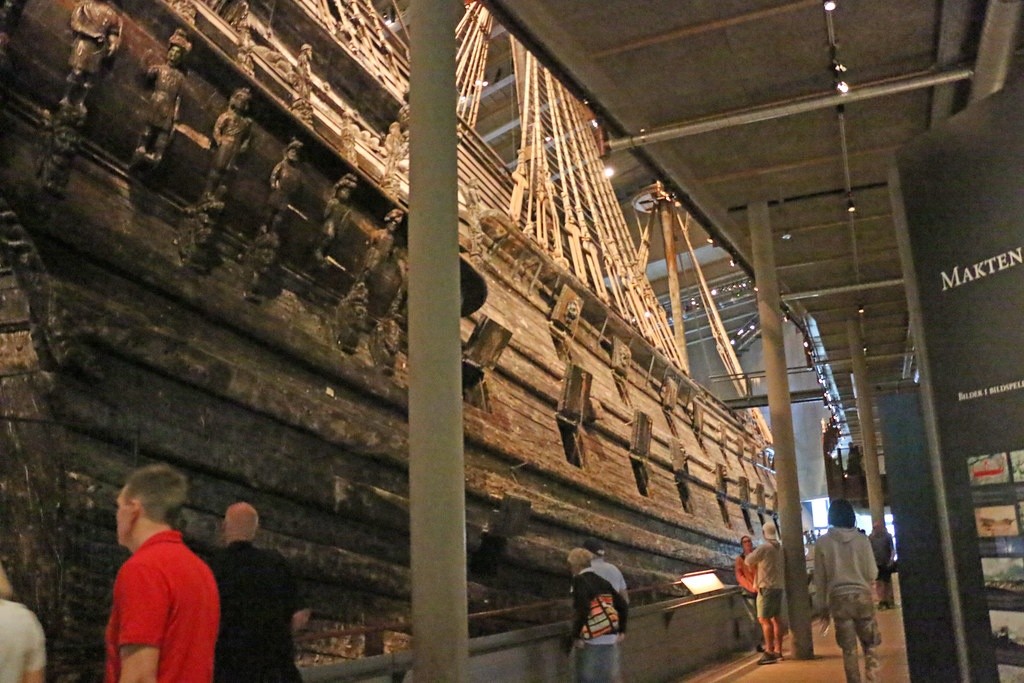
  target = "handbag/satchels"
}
[579,574,619,639]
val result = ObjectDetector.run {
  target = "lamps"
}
[707,236,714,244]
[846,192,855,212]
[836,77,849,93]
[388,5,396,24]
[858,304,864,315]
[729,258,738,267]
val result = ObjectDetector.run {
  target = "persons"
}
[466,178,481,226]
[744,522,786,663]
[0,567,47,683]
[804,529,821,544]
[869,522,895,609]
[202,87,253,206]
[357,209,404,288]
[734,535,765,652]
[135,34,191,156]
[569,539,631,682]
[567,547,628,683]
[846,442,860,475]
[294,43,404,182]
[814,498,882,683]
[313,173,358,269]
[103,462,220,683]
[55,0,124,117]
[212,502,311,683]
[260,139,304,252]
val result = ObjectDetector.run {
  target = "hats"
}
[763,522,777,539]
[584,537,605,556]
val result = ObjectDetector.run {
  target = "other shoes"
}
[757,644,765,652]
[876,601,889,610]
[774,651,784,661]
[757,651,776,664]
